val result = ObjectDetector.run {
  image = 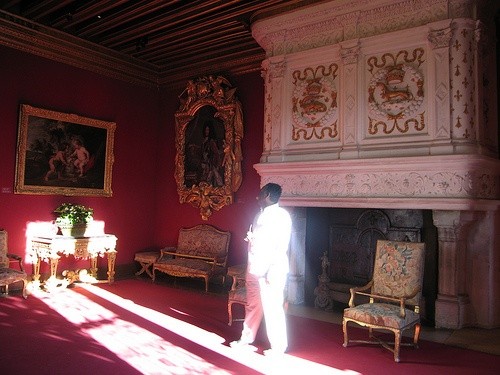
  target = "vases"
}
[58,225,88,238]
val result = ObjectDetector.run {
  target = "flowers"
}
[53,203,94,225]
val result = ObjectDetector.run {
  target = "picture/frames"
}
[173,74,244,220]
[13,104,118,198]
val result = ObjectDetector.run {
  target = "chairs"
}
[342,239,426,363]
[0,229,27,299]
[228,253,248,290]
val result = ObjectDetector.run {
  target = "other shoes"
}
[230,341,251,348]
[263,348,284,356]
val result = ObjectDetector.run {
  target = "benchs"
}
[152,225,232,296]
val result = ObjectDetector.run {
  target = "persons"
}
[229,182,292,356]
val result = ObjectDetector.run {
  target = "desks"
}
[30,234,119,289]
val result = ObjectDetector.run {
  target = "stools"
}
[134,252,173,279]
[227,289,289,327]
